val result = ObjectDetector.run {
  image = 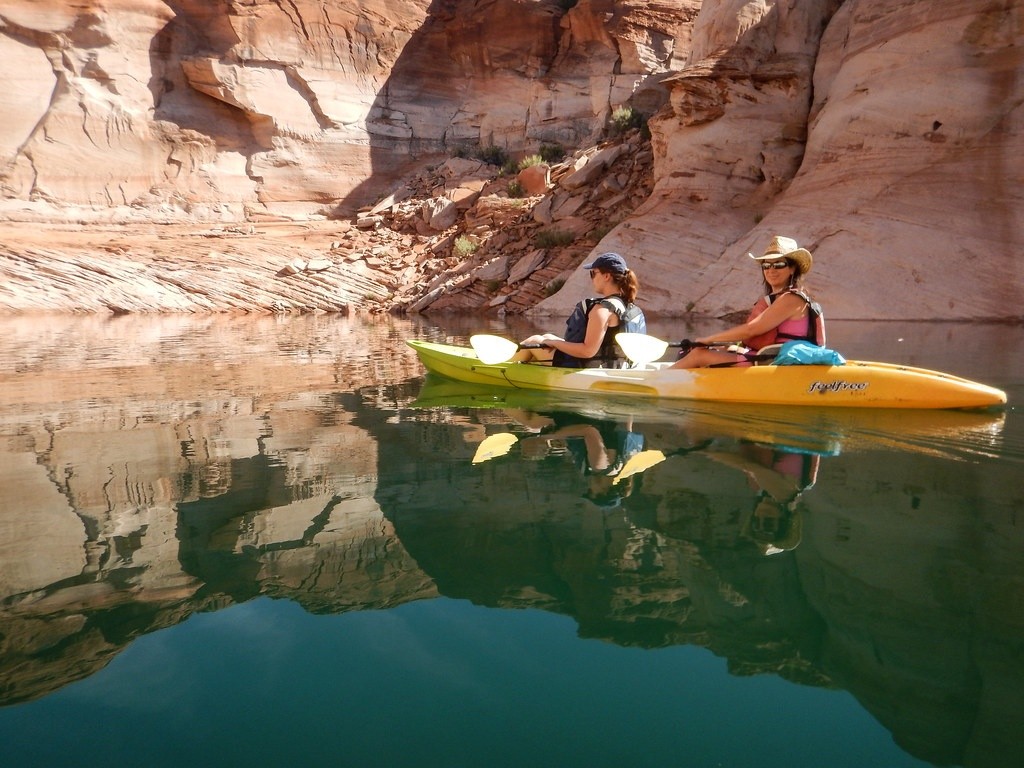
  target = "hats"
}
[579,493,621,508]
[584,253,627,275]
[748,236,812,274]
[739,510,802,556]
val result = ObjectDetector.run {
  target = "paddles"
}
[614,332,741,363]
[472,420,571,465]
[611,436,714,487]
[469,333,558,365]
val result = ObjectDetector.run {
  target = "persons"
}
[512,254,647,370]
[670,236,825,369]
[692,439,819,556]
[503,409,644,509]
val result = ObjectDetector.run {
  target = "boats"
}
[409,370,1006,443]
[408,342,1010,410]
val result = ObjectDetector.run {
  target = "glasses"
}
[750,514,781,532]
[589,270,601,278]
[761,261,788,269]
